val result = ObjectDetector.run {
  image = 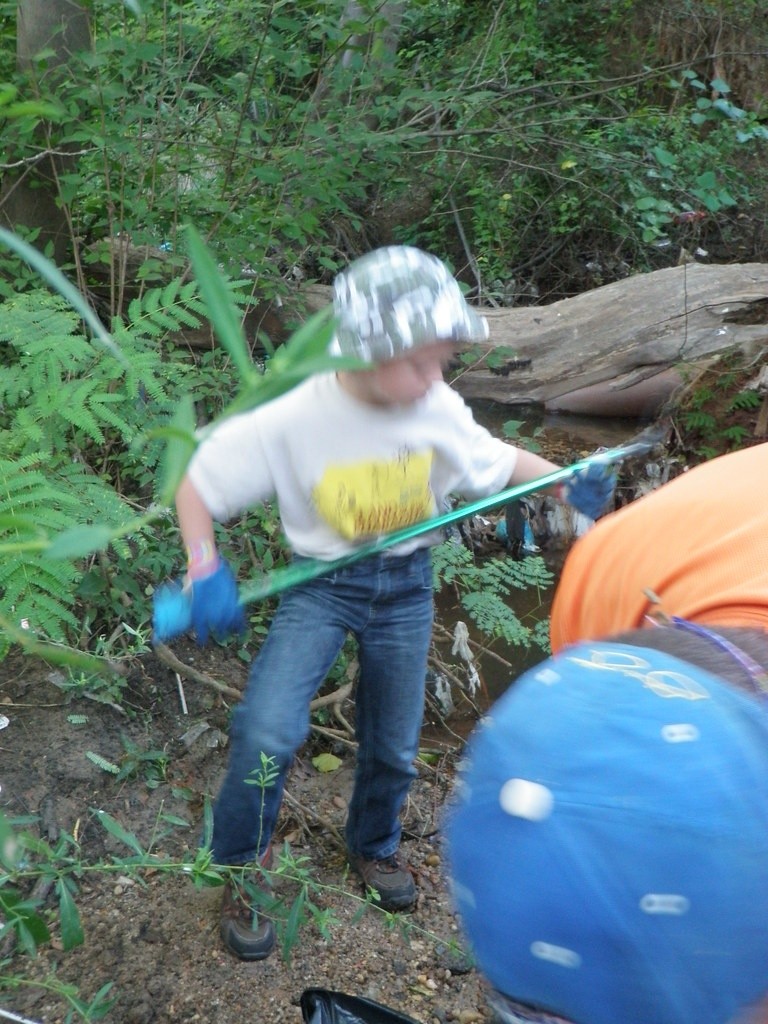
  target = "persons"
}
[176,246,613,961]
[441,441,768,1023]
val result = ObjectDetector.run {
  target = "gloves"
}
[188,560,244,647]
[561,446,618,520]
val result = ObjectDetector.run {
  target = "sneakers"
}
[345,849,416,909]
[221,843,276,959]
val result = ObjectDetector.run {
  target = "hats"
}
[439,643,768,1024]
[327,244,490,367]
